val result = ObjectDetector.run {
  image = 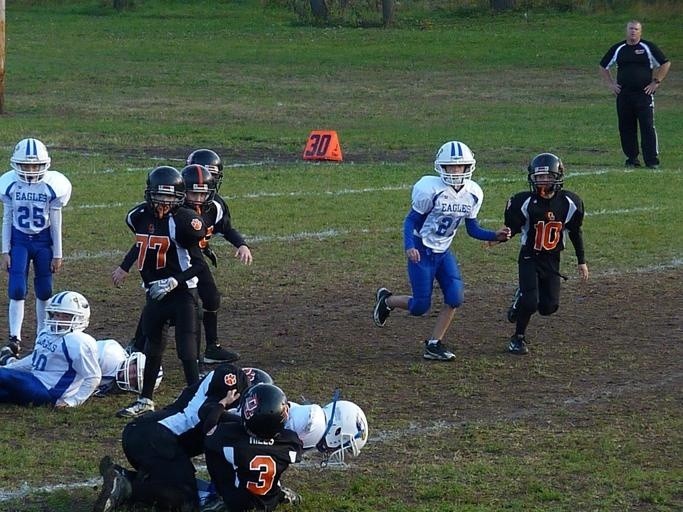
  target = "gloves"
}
[149,277,177,300]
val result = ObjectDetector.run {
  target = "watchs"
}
[653,78,662,84]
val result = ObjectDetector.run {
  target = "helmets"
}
[433,141,477,186]
[42,291,90,336]
[8,138,53,185]
[235,383,291,441]
[181,164,218,213]
[144,165,185,219]
[313,400,369,470]
[526,153,565,194]
[186,148,224,194]
[240,365,274,389]
[115,353,165,396]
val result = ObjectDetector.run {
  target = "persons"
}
[483,153,589,356]
[197,401,369,506]
[0,139,71,357]
[111,166,207,419]
[372,142,511,361]
[0,291,101,409]
[598,21,671,171]
[0,340,164,398]
[125,164,252,364]
[95,365,273,512]
[183,149,231,269]
[194,383,305,512]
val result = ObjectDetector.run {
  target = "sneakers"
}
[280,486,302,505]
[0,346,13,365]
[93,464,132,512]
[647,162,659,168]
[508,335,529,355]
[202,346,242,364]
[508,286,521,323]
[373,287,395,328]
[200,496,227,512]
[116,397,157,421]
[6,339,22,356]
[423,338,457,362]
